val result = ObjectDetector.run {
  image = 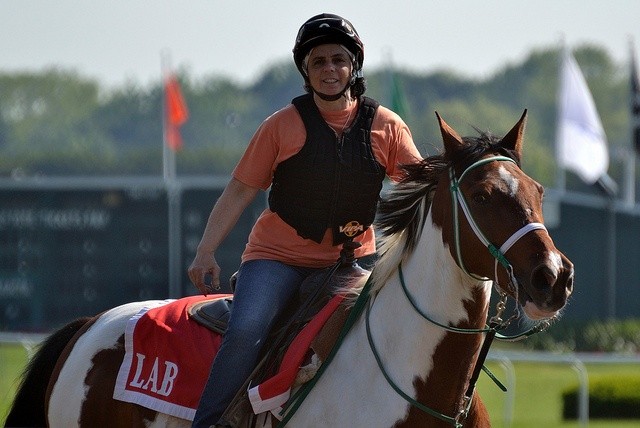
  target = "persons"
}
[186,13,434,427]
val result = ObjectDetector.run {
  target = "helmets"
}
[294,13,364,68]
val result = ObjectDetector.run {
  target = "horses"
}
[3,108,578,428]
